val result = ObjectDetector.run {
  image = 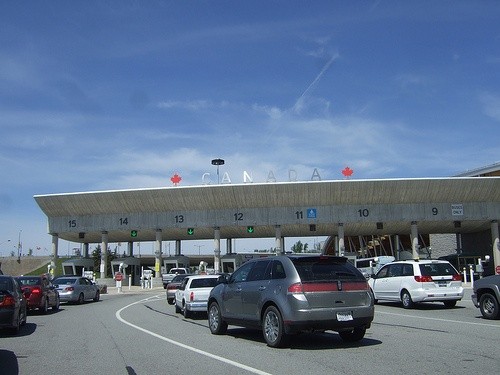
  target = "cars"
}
[166,274,221,319]
[471,274,500,320]
[51,274,100,305]
[16,275,60,314]
[0,275,27,333]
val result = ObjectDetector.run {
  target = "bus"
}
[356,255,396,280]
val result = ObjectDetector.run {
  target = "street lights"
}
[211,158,225,184]
[194,244,206,255]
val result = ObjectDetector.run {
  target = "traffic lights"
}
[187,228,195,235]
[131,230,137,237]
[310,224,316,231]
[376,222,384,229]
[247,226,254,233]
[454,221,461,228]
[79,232,85,238]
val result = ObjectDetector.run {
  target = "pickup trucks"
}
[161,268,195,288]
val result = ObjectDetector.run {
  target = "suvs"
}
[367,257,464,309]
[207,253,375,348]
